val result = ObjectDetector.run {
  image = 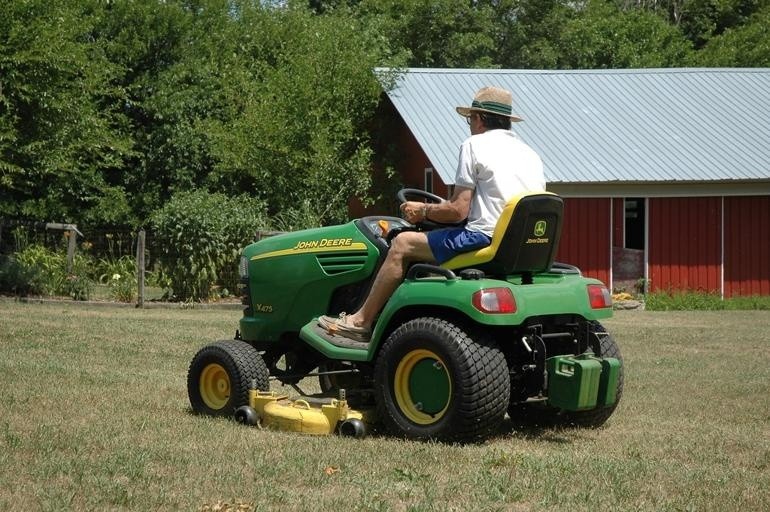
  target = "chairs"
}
[431,191,565,274]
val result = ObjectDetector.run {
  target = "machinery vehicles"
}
[185,188,625,442]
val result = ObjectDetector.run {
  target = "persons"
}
[316,86,548,343]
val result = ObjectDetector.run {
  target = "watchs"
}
[422,202,433,221]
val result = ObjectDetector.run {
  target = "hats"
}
[456,86,524,122]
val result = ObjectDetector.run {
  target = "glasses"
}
[466,114,471,124]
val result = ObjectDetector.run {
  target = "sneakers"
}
[318,312,372,342]
